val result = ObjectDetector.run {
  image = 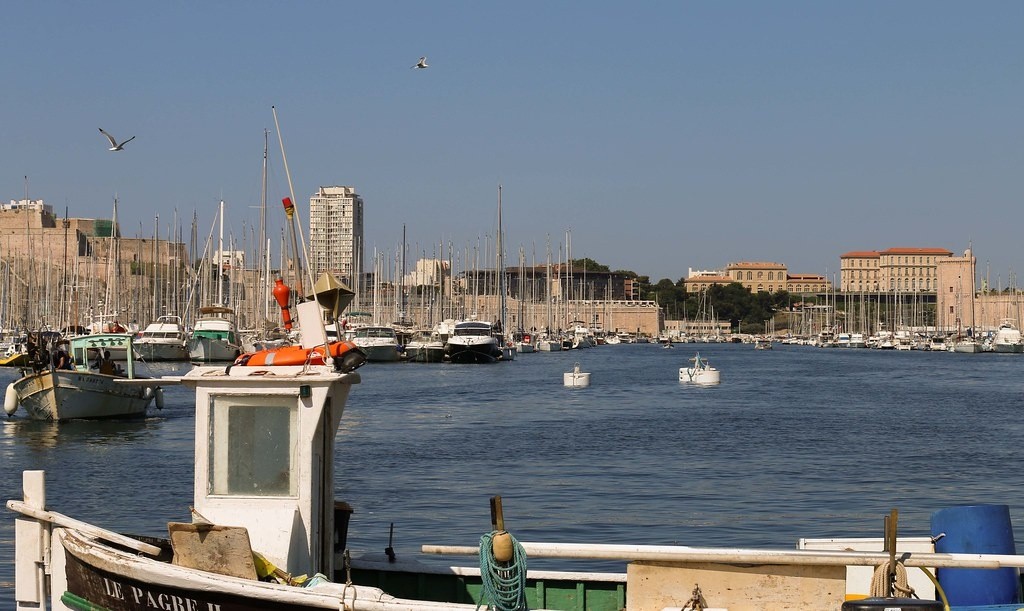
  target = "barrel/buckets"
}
[930,504,1018,606]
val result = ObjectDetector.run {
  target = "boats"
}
[563,362,592,386]
[754,339,772,350]
[235,273,367,371]
[12,332,156,421]
[679,352,719,384]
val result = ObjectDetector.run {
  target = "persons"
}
[52,349,72,370]
[83,351,117,375]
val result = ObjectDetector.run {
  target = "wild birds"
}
[98,128,136,151]
[409,56,429,69]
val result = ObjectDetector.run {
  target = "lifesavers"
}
[234,340,358,367]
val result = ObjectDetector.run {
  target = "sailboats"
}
[240,127,548,362]
[549,226,737,351]
[0,174,243,362]
[956,241,1024,353]
[771,274,957,353]
